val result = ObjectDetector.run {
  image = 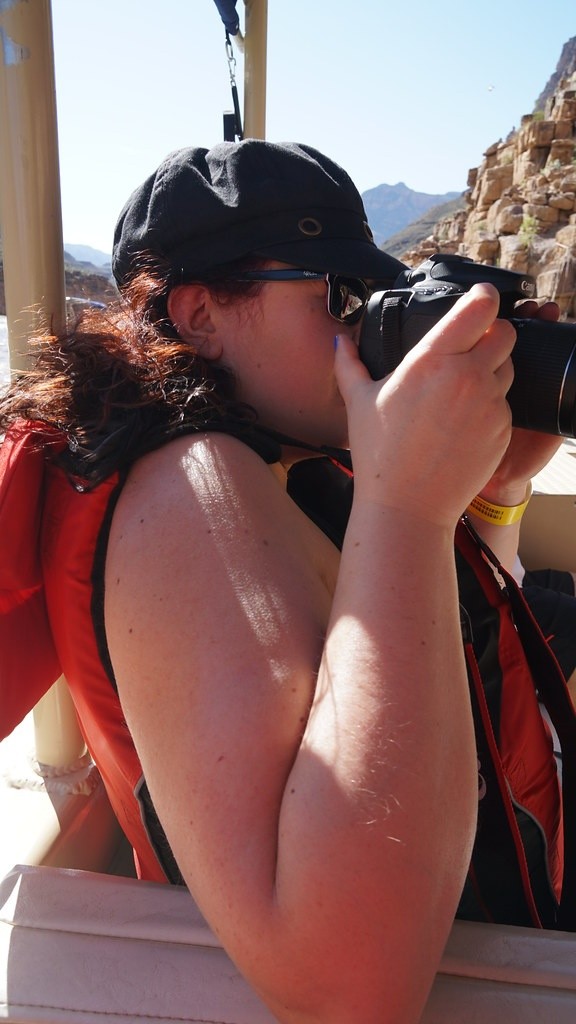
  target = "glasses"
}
[206,267,384,325]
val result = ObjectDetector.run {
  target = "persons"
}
[1,140,576,1024]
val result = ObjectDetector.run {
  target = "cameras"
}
[355,250,576,440]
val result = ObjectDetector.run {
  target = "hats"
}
[112,139,412,308]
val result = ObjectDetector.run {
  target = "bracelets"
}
[468,480,534,526]
